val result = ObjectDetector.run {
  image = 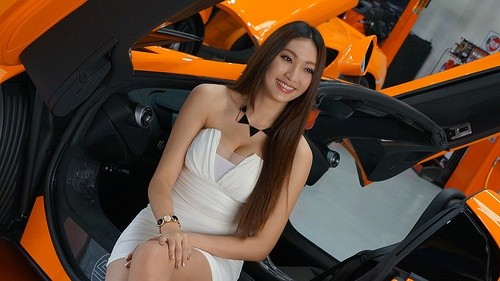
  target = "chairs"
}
[276,188,466,281]
